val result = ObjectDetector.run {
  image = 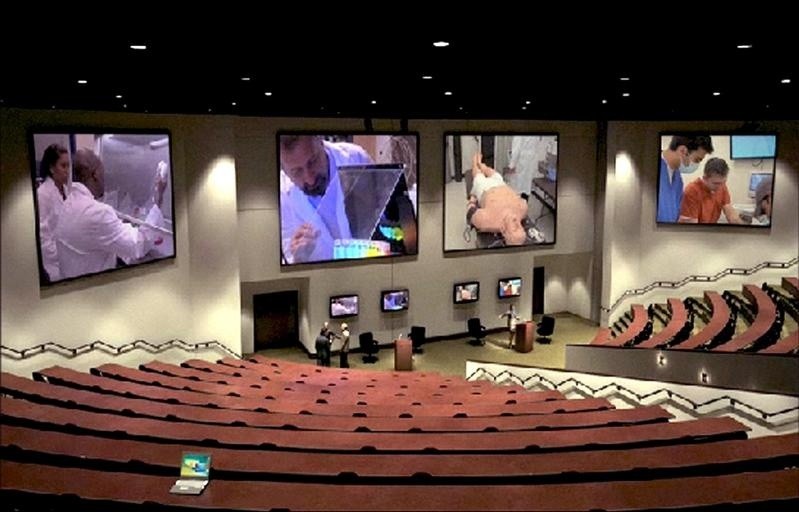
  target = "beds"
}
[464,169,546,249]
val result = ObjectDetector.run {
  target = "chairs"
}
[467,318,488,347]
[407,326,426,353]
[536,315,555,344]
[359,332,380,363]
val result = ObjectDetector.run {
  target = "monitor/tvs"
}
[730,135,776,160]
[497,277,522,299]
[655,129,780,228]
[329,294,360,319]
[276,129,421,266]
[24,127,177,288]
[442,130,560,253]
[453,281,480,304]
[380,288,410,312]
[337,163,416,253]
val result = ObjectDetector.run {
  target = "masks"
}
[678,152,698,174]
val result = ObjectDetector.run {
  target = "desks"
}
[531,178,556,215]
[590,277,799,354]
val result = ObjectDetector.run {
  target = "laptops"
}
[167,450,213,496]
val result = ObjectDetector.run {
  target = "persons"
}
[656,134,714,223]
[316,330,331,367]
[281,135,375,265]
[321,321,335,345]
[336,322,350,368]
[751,177,772,225]
[57,147,168,278]
[679,155,745,225]
[465,150,529,247]
[499,304,519,354]
[36,143,70,283]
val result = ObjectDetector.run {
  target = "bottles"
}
[154,229,164,246]
